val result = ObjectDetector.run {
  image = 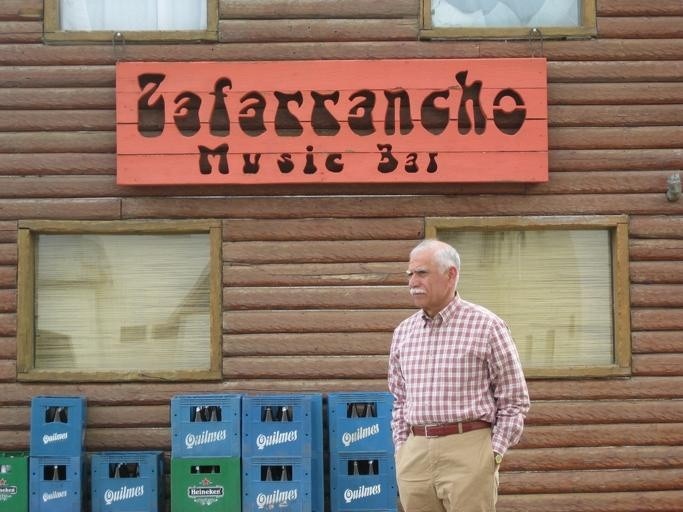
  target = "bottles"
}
[194,405,377,482]
[114,463,139,477]
[50,404,67,423]
[49,465,62,480]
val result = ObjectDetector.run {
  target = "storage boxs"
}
[242,457,324,512]
[1,451,29,512]
[171,395,241,458]
[171,457,241,512]
[29,455,83,512]
[242,396,323,458]
[330,452,397,512]
[91,451,164,512]
[327,394,397,452]
[30,395,86,457]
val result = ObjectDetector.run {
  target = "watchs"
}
[494,454,503,465]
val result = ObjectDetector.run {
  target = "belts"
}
[409,420,491,437]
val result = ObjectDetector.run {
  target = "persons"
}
[387,240,531,512]
